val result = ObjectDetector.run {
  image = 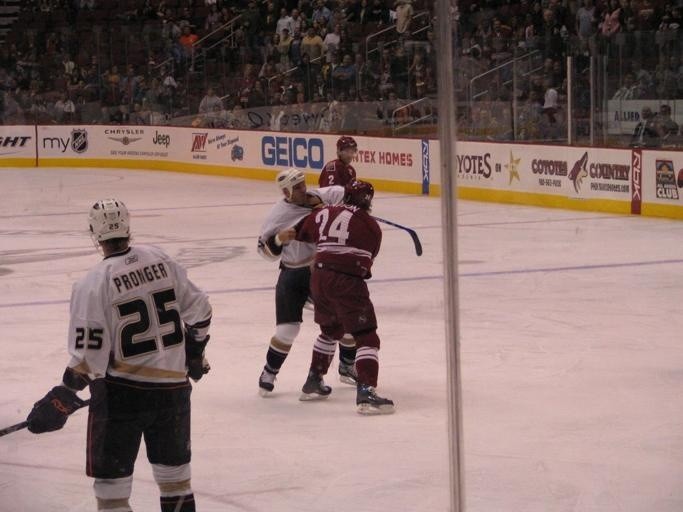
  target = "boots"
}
[259,359,393,405]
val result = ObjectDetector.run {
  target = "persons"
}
[296,178,394,406]
[28,199,212,511]
[453,1,682,149]
[318,137,357,186]
[259,168,363,391]
[1,1,436,136]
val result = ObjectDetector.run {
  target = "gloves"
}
[27,387,90,434]
[184,333,209,380]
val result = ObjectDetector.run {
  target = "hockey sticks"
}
[371,215,422,257]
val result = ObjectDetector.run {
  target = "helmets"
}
[276,168,304,191]
[88,198,128,256]
[344,180,373,206]
[337,137,356,150]
